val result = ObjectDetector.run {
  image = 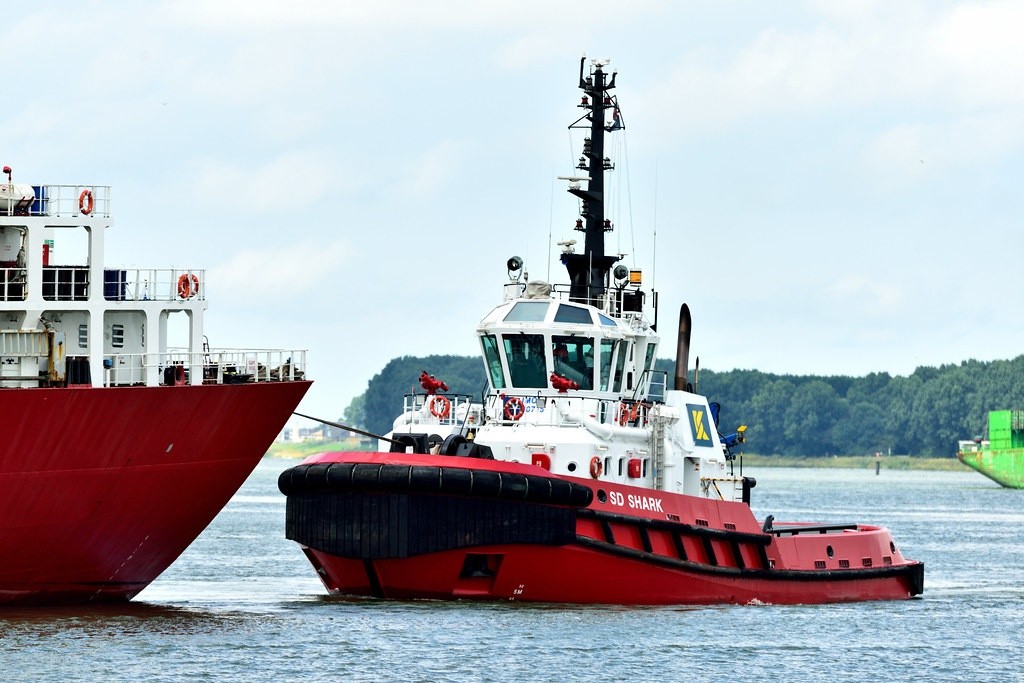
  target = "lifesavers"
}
[177,273,199,300]
[589,456,603,478]
[79,189,94,214]
[618,402,629,426]
[505,398,525,419]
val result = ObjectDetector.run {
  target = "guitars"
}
[429,396,450,418]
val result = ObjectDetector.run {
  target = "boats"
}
[278,56,924,605]
[0,166,315,601]
[956,409,1024,490]
